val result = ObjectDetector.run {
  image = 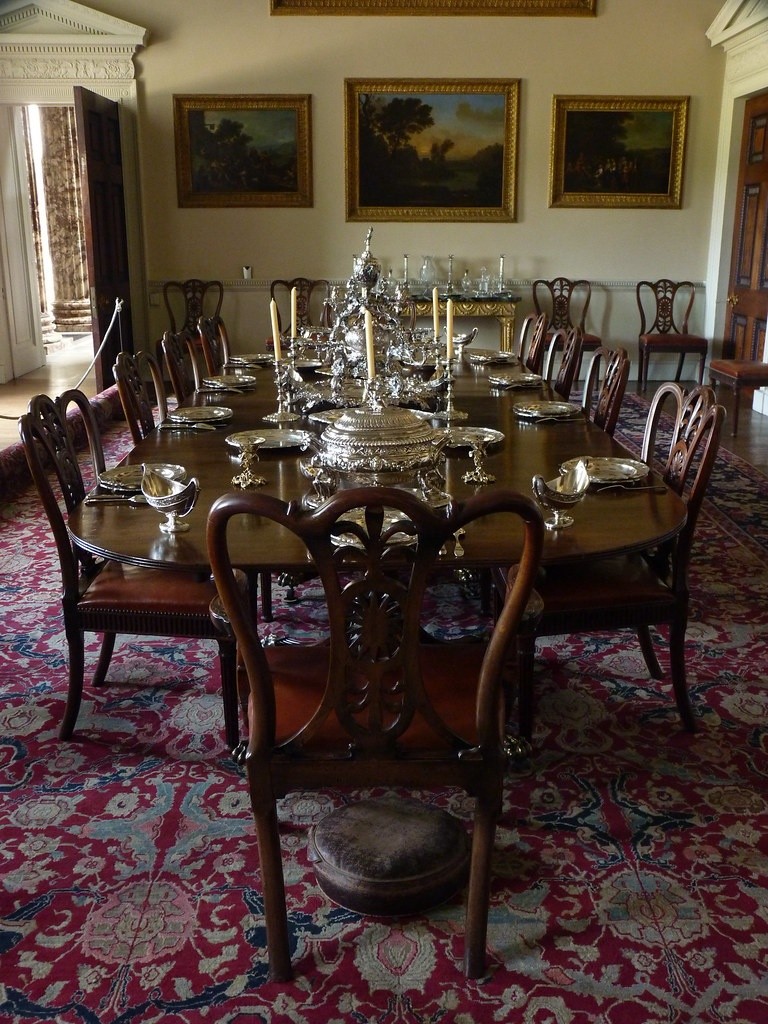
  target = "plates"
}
[513,401,581,417]
[559,456,650,483]
[202,375,257,388]
[226,428,309,449]
[167,406,233,422]
[98,464,187,492]
[469,351,514,361]
[436,427,505,447]
[229,353,274,364]
[487,373,541,385]
[330,507,418,549]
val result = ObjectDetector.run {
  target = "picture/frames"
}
[172,94,313,209]
[342,77,519,223]
[548,95,691,209]
[269,0,599,18]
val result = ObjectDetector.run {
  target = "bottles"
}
[418,255,488,292]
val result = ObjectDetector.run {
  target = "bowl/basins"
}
[282,358,322,374]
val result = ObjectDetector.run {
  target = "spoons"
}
[196,388,243,393]
[535,417,585,423]
[453,528,466,556]
[84,495,147,504]
[505,384,544,390]
[483,361,513,365]
[597,485,666,492]
[223,363,262,369]
[158,423,216,431]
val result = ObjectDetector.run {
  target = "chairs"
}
[19,277,730,982]
[709,359,768,436]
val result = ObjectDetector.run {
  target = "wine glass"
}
[140,462,199,532]
[443,327,478,363]
[531,460,589,529]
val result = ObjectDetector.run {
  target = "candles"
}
[269,298,281,361]
[447,299,454,359]
[365,310,375,379]
[291,287,297,337]
[433,287,440,336]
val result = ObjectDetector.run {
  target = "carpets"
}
[0,380,767,1024]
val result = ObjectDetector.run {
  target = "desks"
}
[67,344,688,646]
[347,294,522,353]
